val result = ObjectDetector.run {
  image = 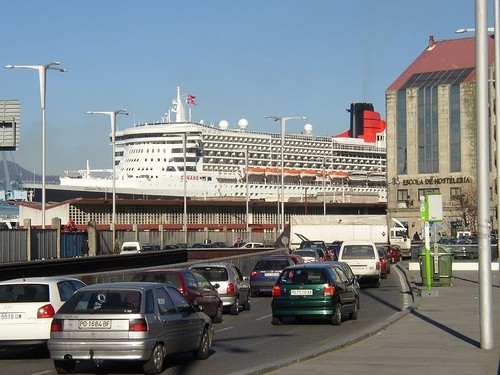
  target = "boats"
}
[283,169,300,176]
[265,167,282,176]
[327,171,349,179]
[301,170,317,177]
[246,167,266,175]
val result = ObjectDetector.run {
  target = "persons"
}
[413,231,420,241]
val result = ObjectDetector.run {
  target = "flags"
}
[187,95,197,106]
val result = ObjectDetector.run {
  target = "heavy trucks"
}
[287,213,411,250]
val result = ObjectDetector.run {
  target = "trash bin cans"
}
[419,246,456,287]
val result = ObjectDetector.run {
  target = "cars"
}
[250,257,302,297]
[375,243,402,279]
[141,242,266,251]
[188,263,251,316]
[436,228,499,261]
[267,238,344,266]
[0,276,88,358]
[129,268,223,325]
[46,281,215,375]
[308,261,362,297]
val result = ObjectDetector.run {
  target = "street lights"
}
[162,131,204,243]
[2,61,67,228]
[264,115,308,233]
[86,108,131,255]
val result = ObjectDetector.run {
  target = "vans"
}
[337,240,383,288]
[119,241,142,255]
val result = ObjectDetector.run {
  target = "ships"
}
[271,263,359,326]
[19,84,386,225]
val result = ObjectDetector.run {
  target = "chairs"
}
[294,274,305,283]
[104,293,121,306]
